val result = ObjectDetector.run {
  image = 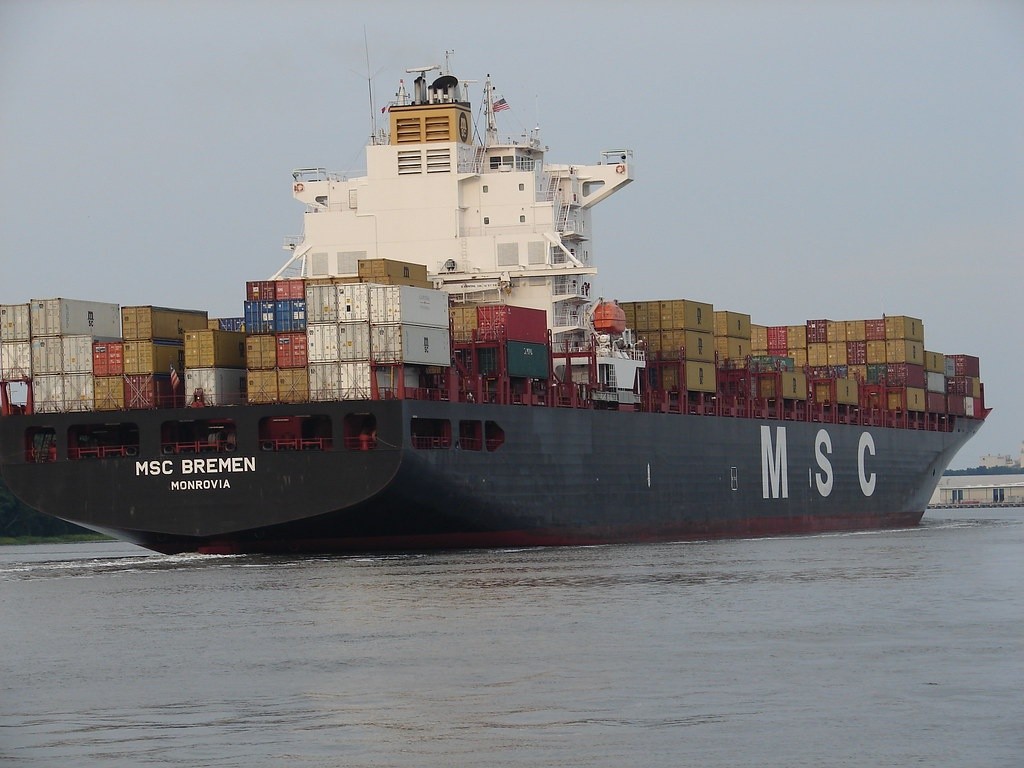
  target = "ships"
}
[0,48,995,559]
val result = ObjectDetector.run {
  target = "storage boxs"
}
[619,299,980,418]
[0,258,552,415]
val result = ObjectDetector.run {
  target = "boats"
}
[593,302,626,333]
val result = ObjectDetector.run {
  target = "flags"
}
[492,98,510,112]
[170,364,180,389]
